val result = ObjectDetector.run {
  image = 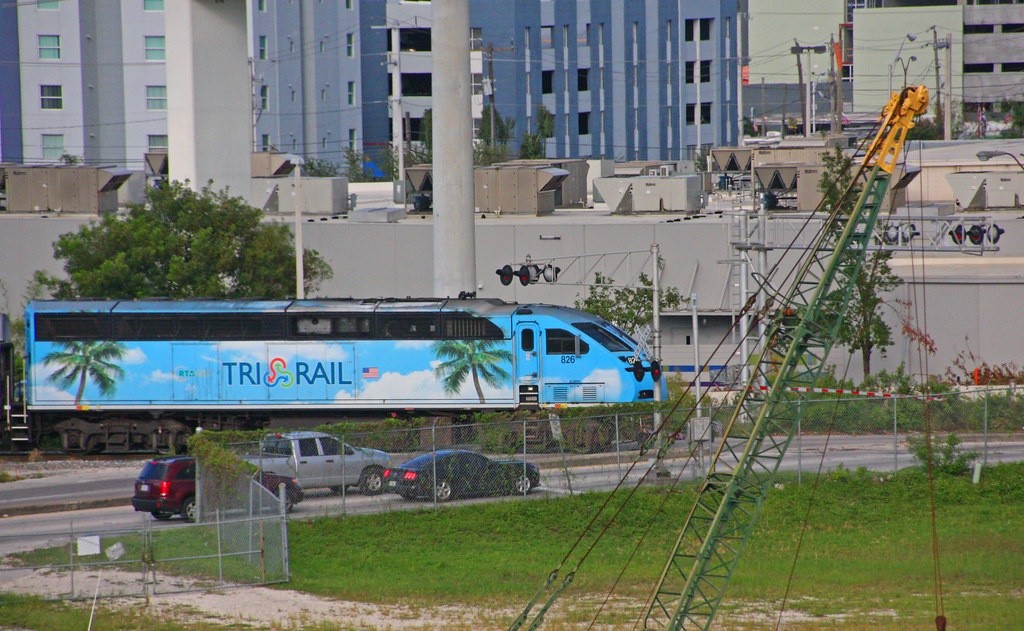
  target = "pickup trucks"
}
[241,430,395,495]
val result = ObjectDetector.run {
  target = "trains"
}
[1,297,661,456]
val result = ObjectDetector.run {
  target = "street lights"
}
[889,33,917,97]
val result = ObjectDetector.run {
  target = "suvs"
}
[133,456,305,524]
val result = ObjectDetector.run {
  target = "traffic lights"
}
[496,265,514,286]
[624,362,644,382]
[644,360,661,382]
[513,266,530,287]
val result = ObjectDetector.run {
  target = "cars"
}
[382,450,540,502]
